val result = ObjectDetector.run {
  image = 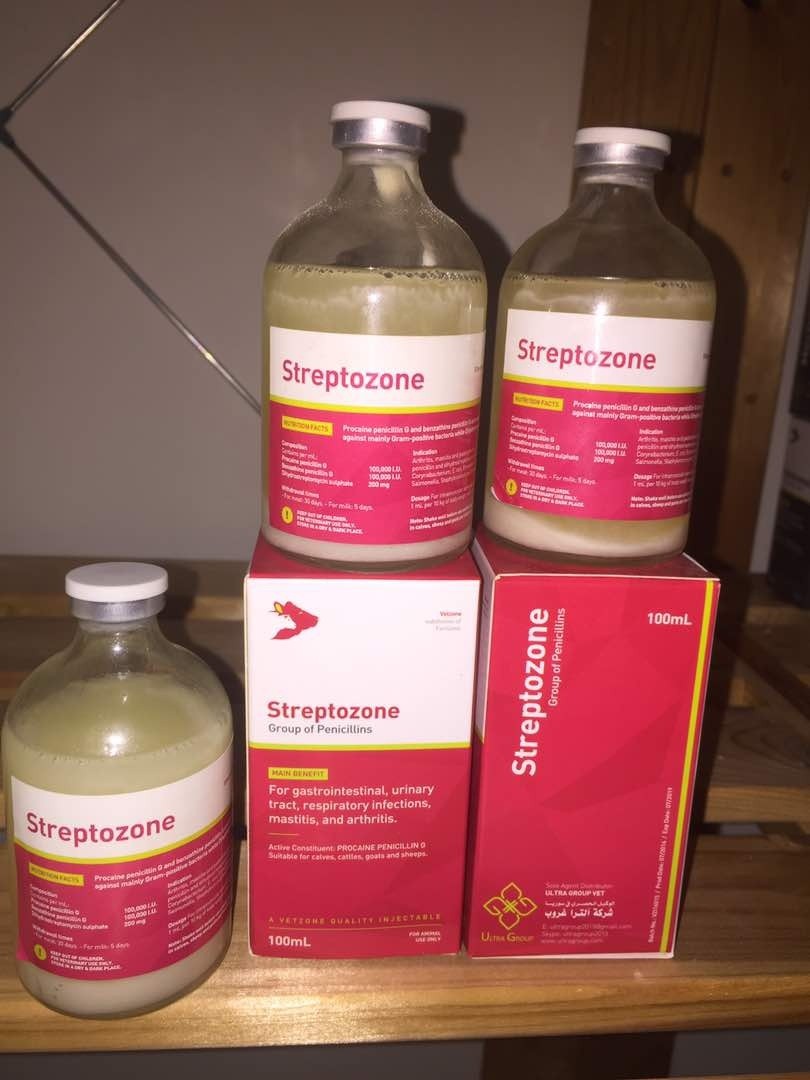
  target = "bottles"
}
[482,123,719,563]
[260,104,486,571]
[1,562,234,1018]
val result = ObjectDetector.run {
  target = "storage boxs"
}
[242,520,721,959]
